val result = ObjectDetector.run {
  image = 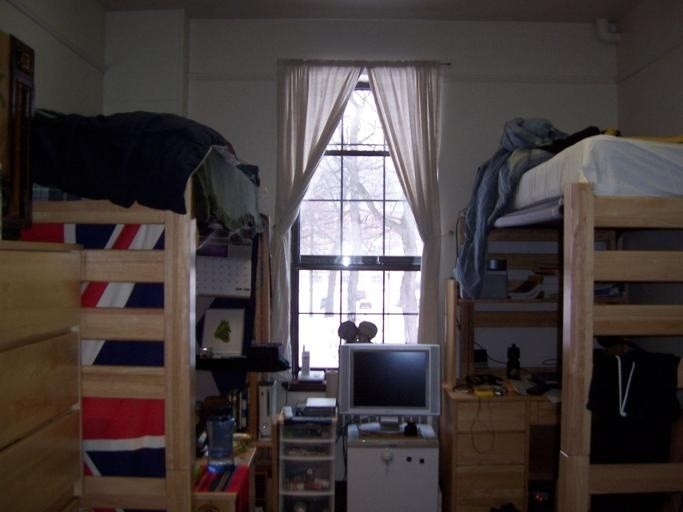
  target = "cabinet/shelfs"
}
[279,406,336,511]
[1,238,86,510]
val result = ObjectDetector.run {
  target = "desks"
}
[194,428,255,511]
[440,375,562,511]
[347,424,439,512]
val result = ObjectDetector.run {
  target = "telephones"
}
[298,345,325,382]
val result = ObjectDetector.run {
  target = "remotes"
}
[283,406,293,420]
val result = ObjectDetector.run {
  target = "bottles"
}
[481,258,507,299]
[505,343,521,380]
[301,351,310,376]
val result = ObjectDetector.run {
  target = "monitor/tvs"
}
[337,343,442,436]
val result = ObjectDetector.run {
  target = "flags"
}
[16,221,167,512]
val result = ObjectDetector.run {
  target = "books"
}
[304,397,337,416]
[190,379,277,511]
[507,266,563,302]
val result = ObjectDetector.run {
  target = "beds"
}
[445,136,682,510]
[18,177,272,510]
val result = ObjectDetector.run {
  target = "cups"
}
[204,411,236,474]
